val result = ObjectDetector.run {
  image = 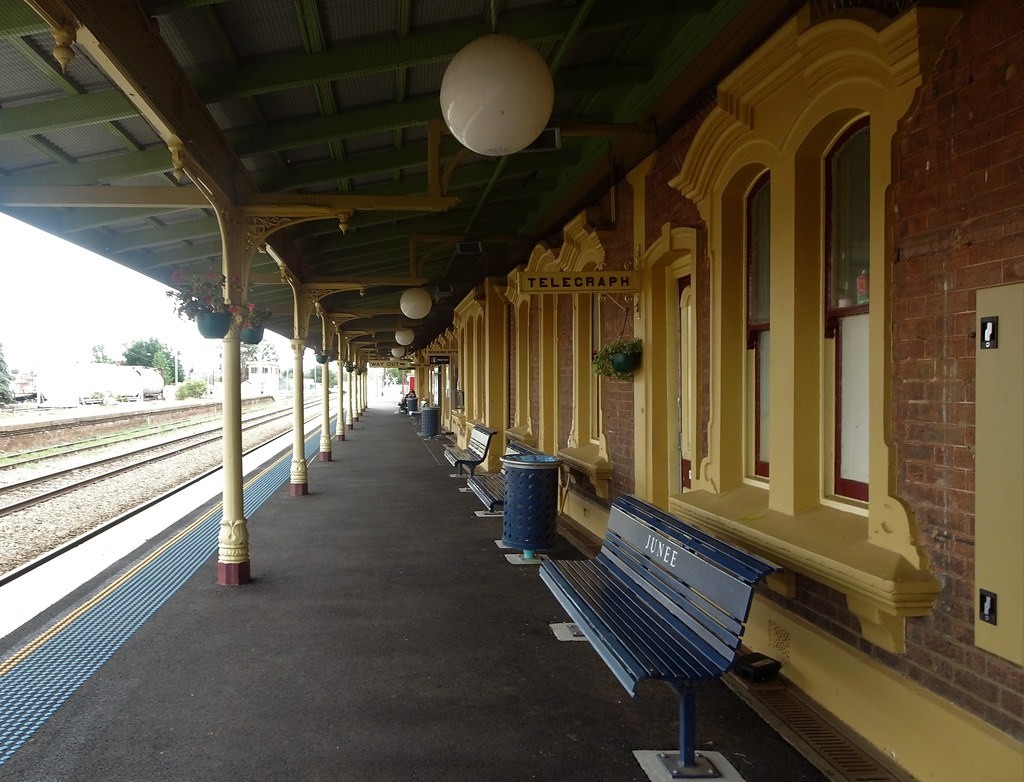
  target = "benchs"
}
[409,400,427,425]
[468,442,547,547]
[539,493,783,782]
[444,423,499,493]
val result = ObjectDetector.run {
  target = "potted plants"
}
[591,336,643,382]
[345,360,354,373]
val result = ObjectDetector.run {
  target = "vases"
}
[316,355,329,364]
[240,323,265,344]
[196,311,233,338]
[357,371,361,375]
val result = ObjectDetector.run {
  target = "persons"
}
[394,389,416,415]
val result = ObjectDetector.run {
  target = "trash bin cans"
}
[422,408,440,435]
[406,398,417,415]
[500,455,563,549]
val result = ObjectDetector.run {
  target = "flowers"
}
[313,345,340,362]
[357,363,367,373]
[242,303,273,329]
[166,271,244,322]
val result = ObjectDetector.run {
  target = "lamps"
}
[395,328,414,346]
[390,357,400,363]
[399,288,432,320]
[439,0,555,157]
[391,346,405,357]
